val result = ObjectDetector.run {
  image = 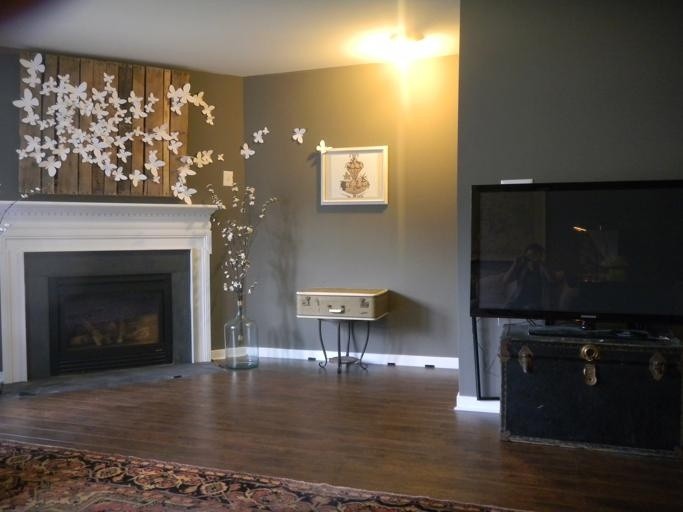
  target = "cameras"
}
[518,256,529,264]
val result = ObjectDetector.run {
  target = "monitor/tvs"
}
[469,178,682,340]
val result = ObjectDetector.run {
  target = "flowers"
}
[207,183,281,342]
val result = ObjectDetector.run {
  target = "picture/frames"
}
[319,144,389,208]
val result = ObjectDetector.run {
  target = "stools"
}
[318,319,370,372]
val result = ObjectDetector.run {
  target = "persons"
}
[501,244,550,311]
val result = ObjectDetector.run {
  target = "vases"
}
[223,294,260,371]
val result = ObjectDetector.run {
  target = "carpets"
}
[1,441,524,512]
